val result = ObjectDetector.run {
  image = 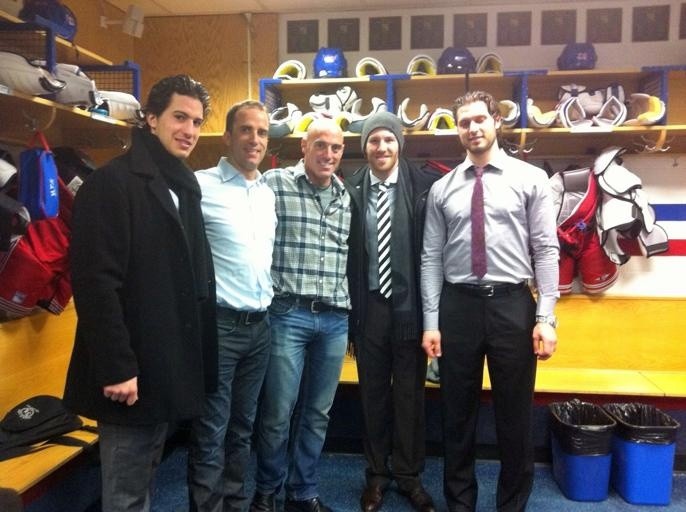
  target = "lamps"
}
[100,3,144,38]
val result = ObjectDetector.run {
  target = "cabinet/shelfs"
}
[0,9,141,130]
[259,65,686,160]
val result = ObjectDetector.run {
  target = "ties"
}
[472,168,487,278]
[377,182,391,298]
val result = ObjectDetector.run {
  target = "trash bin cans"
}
[605,402,680,505]
[548,402,616,501]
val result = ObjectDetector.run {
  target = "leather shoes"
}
[283,500,332,511]
[361,479,382,508]
[406,483,433,509]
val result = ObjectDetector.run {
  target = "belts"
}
[217,309,270,324]
[451,282,526,297]
[283,293,336,313]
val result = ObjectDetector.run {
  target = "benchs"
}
[1,308,101,509]
[340,295,685,475]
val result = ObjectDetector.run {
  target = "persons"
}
[420,92,562,512]
[66,74,215,512]
[347,112,445,512]
[189,101,280,512]
[250,119,351,512]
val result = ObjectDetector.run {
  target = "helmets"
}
[557,44,598,70]
[22,4,77,42]
[313,48,347,79]
[439,46,476,74]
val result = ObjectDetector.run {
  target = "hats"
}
[362,112,406,150]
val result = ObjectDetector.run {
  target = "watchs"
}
[534,315,559,327]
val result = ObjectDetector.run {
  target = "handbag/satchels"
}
[19,131,56,224]
[14,395,100,459]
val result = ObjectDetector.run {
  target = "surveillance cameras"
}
[122,5,145,39]
